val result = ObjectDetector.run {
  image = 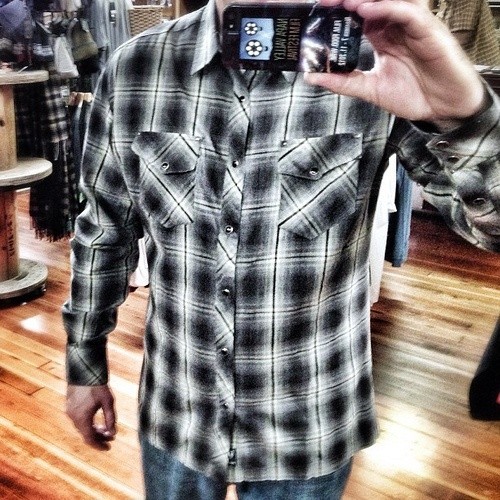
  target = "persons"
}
[59,0,500,500]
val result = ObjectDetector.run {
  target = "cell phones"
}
[222,3,364,75]
